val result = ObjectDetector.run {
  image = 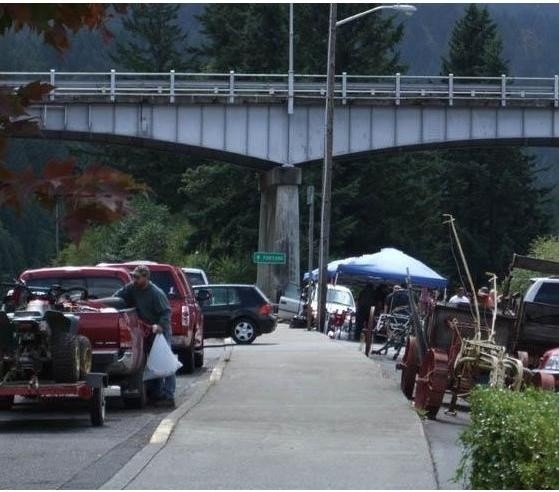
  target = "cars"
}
[96,261,278,375]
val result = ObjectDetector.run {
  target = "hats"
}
[458,284,464,290]
[478,287,489,297]
[130,265,150,276]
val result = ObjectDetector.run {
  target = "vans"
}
[278,282,357,326]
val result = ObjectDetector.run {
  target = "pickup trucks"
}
[13,266,147,408]
[425,278,559,374]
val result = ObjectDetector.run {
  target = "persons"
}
[448,287,470,304]
[358,282,376,322]
[383,285,401,336]
[112,265,176,407]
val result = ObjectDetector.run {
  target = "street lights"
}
[315,1,415,333]
